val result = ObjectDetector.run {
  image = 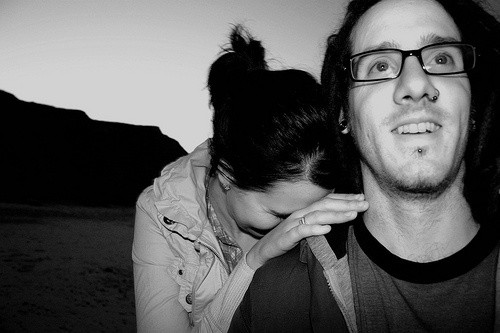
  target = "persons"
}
[129,24,370,333]
[228,0,500,333]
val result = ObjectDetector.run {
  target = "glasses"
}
[343,42,477,82]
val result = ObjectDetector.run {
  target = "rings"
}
[298,216,308,225]
